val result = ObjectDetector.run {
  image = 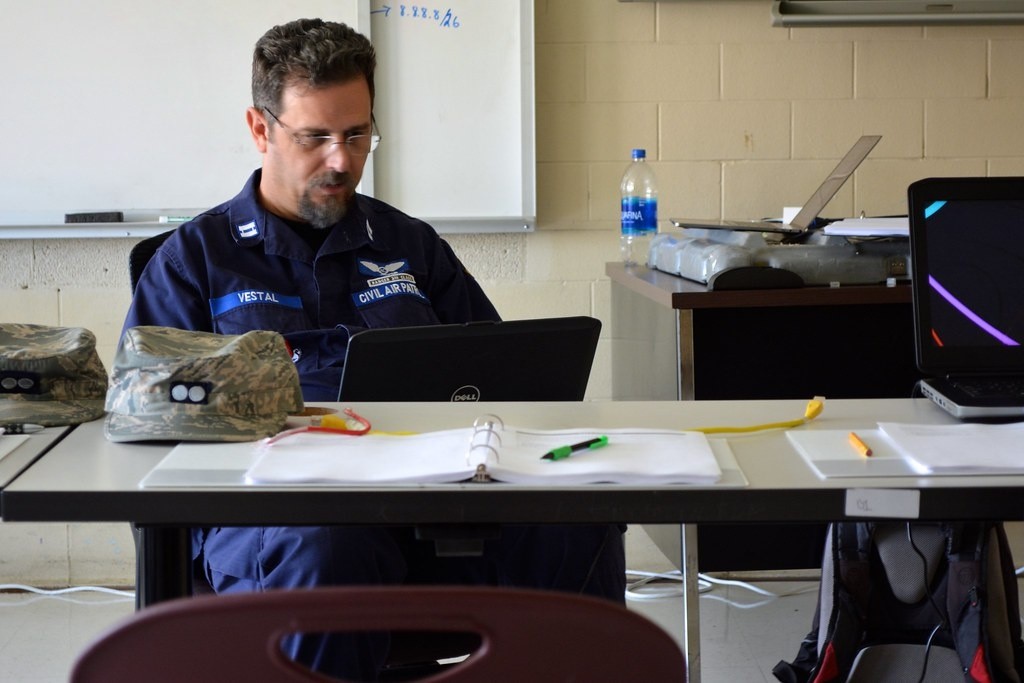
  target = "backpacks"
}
[770,515,1024,683]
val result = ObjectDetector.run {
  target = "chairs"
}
[68,585,687,683]
[129,229,217,611]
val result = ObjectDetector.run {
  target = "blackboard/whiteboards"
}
[1,0,541,241]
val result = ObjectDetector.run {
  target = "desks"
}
[0,397,1024,525]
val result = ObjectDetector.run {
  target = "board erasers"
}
[65,211,124,224]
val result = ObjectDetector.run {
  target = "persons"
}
[117,17,627,683]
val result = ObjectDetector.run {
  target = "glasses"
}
[260,105,383,158]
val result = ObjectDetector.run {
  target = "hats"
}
[102,325,306,443]
[0,322,108,426]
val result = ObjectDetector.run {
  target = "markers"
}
[158,215,196,224]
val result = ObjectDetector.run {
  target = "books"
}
[783,422,1024,480]
[822,216,910,235]
[243,416,722,486]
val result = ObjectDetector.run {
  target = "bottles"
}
[619,149,658,266]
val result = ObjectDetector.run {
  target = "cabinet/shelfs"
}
[601,261,932,580]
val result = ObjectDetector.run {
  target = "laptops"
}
[337,316,602,406]
[907,176,1024,418]
[670,135,883,231]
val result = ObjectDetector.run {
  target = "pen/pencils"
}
[540,436,609,461]
[849,430,873,457]
[0,422,46,434]
